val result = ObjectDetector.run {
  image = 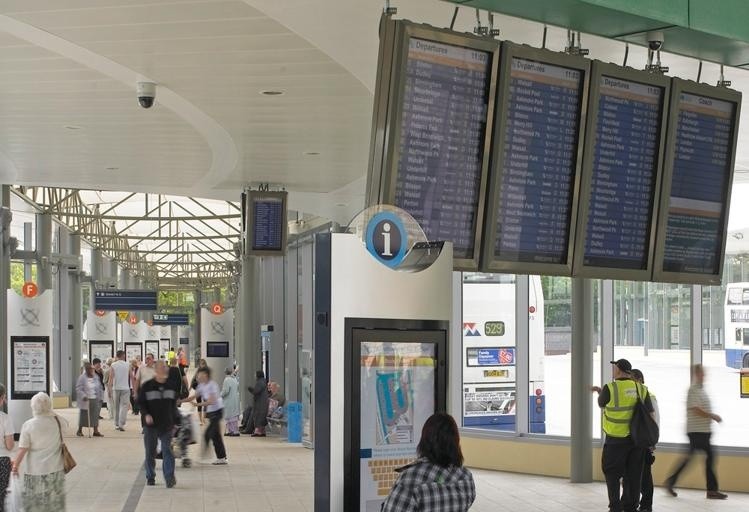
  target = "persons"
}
[238,371,285,437]
[0,385,70,511]
[381,414,476,512]
[663,363,728,499]
[76,349,240,489]
[598,358,656,512]
[591,369,660,512]
[302,368,312,436]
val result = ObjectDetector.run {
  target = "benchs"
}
[267,400,291,435]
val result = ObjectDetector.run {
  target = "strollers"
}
[157,400,196,468]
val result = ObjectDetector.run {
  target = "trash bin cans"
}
[288,402,302,443]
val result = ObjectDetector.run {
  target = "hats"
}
[610,359,631,374]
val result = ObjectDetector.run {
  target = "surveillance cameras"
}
[136,83,156,108]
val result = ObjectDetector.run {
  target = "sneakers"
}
[147,478,155,485]
[707,490,727,499]
[77,430,84,436]
[212,457,228,465]
[224,431,240,436]
[166,476,176,488]
[114,425,124,431]
[667,487,677,497]
[93,431,104,436]
[251,433,267,437]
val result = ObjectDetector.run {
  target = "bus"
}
[462,272,545,435]
[724,282,749,369]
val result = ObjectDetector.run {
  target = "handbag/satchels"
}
[192,378,198,389]
[62,443,77,474]
[631,400,659,446]
[271,410,282,419]
[180,382,188,399]
